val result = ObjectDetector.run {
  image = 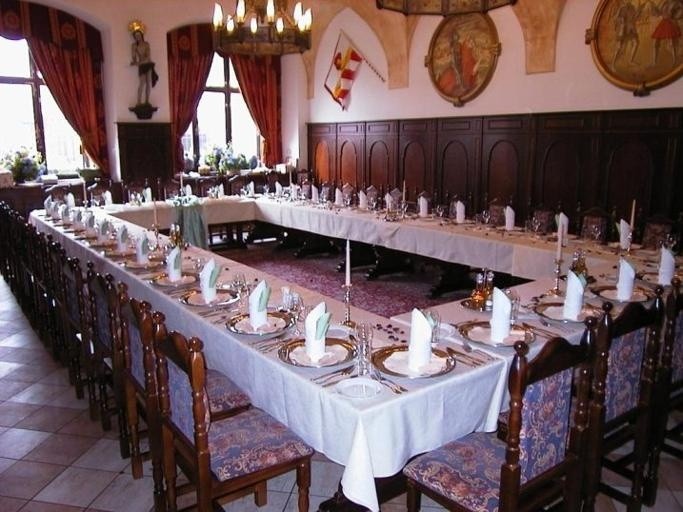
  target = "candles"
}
[154,198,158,225]
[345,238,351,285]
[630,200,636,229]
[557,213,562,259]
[403,180,406,202]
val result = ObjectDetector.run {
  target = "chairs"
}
[197,177,228,244]
[635,206,682,253]
[292,179,442,217]
[121,179,148,204]
[86,179,112,208]
[579,285,664,512]
[576,201,620,243]
[444,191,472,219]
[87,261,152,459]
[157,176,184,200]
[117,281,252,480]
[403,317,598,512]
[483,192,513,226]
[603,277,683,507]
[153,311,316,512]
[229,175,251,243]
[0,200,95,400]
[40,183,73,208]
[524,196,562,232]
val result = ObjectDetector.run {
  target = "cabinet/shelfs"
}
[0,179,85,215]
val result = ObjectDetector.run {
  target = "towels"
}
[555,212,569,238]
[304,300,333,363]
[218,183,224,199]
[615,219,632,250]
[44,194,52,217]
[62,205,73,225]
[199,256,224,304]
[408,307,438,374]
[166,244,182,282]
[101,190,112,207]
[335,187,343,205]
[503,205,515,230]
[64,192,75,208]
[85,215,96,238]
[249,181,254,195]
[97,218,111,241]
[117,225,128,252]
[186,184,192,196]
[563,269,587,321]
[52,200,61,219]
[291,182,297,198]
[275,181,282,195]
[617,256,635,302]
[491,286,512,344]
[357,190,368,209]
[453,200,465,223]
[142,187,152,203]
[72,209,83,229]
[312,184,318,202]
[135,230,151,265]
[417,196,427,217]
[385,193,393,208]
[658,244,675,286]
[248,279,272,331]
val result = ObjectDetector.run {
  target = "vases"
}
[14,176,26,186]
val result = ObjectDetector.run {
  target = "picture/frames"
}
[425,12,502,107]
[586,0,683,96]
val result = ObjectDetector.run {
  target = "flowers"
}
[1,146,46,181]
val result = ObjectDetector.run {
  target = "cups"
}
[358,339,372,376]
[396,203,402,215]
[432,208,437,220]
[510,297,519,325]
[240,188,245,197]
[524,220,531,236]
[476,214,481,228]
[432,324,440,343]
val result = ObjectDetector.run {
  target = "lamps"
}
[211,1,312,56]
[375,0,517,17]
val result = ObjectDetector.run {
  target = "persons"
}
[130,29,158,105]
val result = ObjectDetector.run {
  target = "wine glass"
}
[482,211,490,225]
[438,206,443,217]
[401,201,407,214]
[422,307,439,330]
[345,193,351,205]
[532,216,539,234]
[243,185,247,196]
[357,322,372,343]
[502,290,517,305]
[265,185,269,193]
[370,195,377,208]
[233,272,244,299]
[592,225,601,239]
[193,258,205,280]
[667,233,676,252]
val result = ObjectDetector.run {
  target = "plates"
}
[553,231,580,241]
[278,336,354,368]
[642,273,659,287]
[535,300,603,324]
[592,285,653,303]
[106,250,137,259]
[497,225,522,232]
[461,299,493,311]
[373,345,455,377]
[228,310,291,335]
[326,324,353,340]
[126,258,162,270]
[436,322,456,340]
[454,218,474,226]
[462,321,534,348]
[180,289,241,308]
[150,271,200,289]
[608,242,643,251]
[335,377,383,401]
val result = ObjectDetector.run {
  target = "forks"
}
[317,366,354,385]
[369,375,400,395]
[375,371,409,392]
[448,350,477,369]
[250,332,288,345]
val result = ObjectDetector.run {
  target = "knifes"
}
[322,375,358,388]
[311,367,346,381]
[447,347,483,366]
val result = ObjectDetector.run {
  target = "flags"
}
[324,31,365,111]
